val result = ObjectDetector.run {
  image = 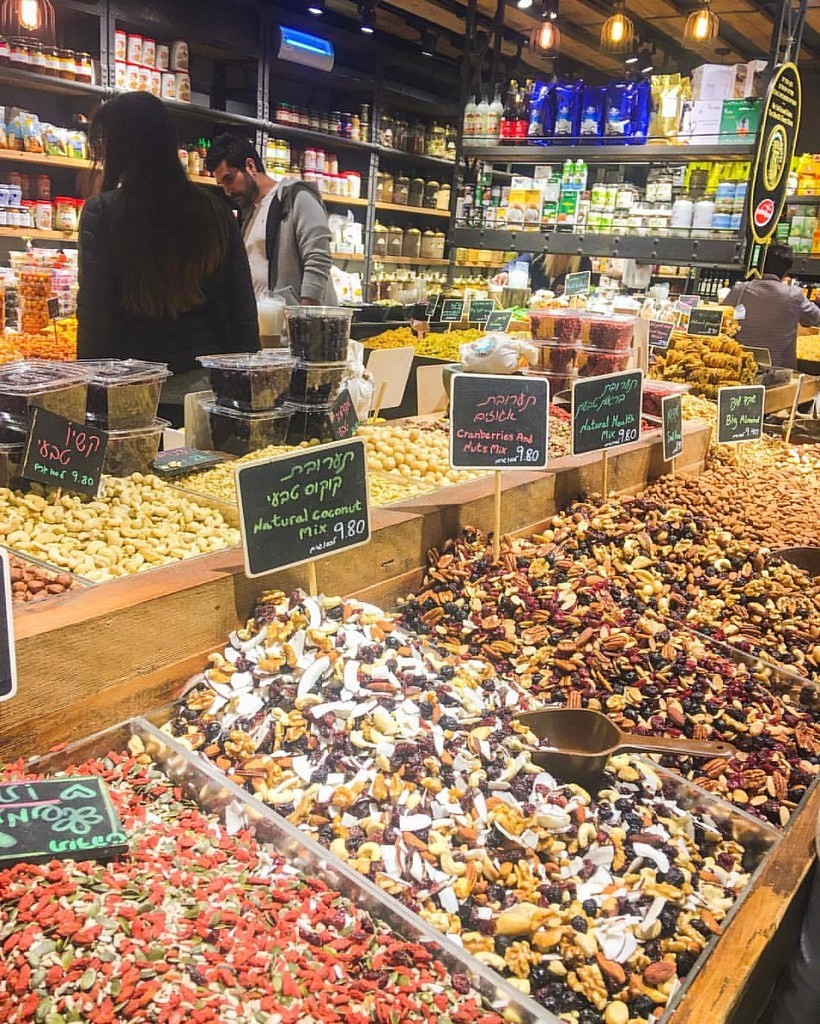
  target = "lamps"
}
[598,0,634,55]
[0,0,56,61]
[530,0,561,60]
[680,0,719,50]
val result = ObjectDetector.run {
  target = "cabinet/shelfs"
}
[0,0,465,315]
[448,79,773,316]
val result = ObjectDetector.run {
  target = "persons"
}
[620,257,653,296]
[718,243,820,371]
[73,92,260,449]
[204,134,338,347]
[491,253,592,298]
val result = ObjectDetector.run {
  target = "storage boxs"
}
[479,250,492,262]
[729,64,746,98]
[787,236,812,254]
[799,180,820,189]
[679,100,723,144]
[720,99,765,145]
[790,216,815,238]
[744,60,768,97]
[798,189,820,195]
[449,248,468,261]
[492,251,504,262]
[468,249,479,261]
[504,253,516,262]
[691,64,731,100]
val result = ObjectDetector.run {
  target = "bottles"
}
[460,79,534,145]
[351,114,361,140]
[692,270,735,296]
